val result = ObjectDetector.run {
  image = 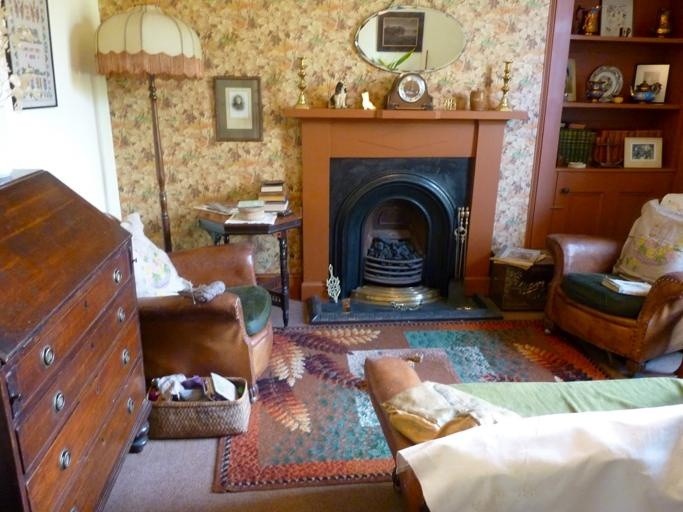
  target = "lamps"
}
[94,4,203,251]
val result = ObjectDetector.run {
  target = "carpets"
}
[211,320,624,493]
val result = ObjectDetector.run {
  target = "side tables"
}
[197,210,303,327]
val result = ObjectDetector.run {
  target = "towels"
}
[181,278,227,303]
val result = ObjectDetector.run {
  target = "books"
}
[603,279,653,296]
[221,179,291,230]
[490,246,547,270]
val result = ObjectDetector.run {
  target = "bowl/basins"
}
[237,206,265,221]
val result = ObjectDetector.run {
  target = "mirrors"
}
[354,5,468,75]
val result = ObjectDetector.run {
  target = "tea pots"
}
[629,81,658,102]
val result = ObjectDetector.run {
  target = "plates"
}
[587,64,624,102]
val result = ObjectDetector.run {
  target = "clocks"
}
[388,74,433,111]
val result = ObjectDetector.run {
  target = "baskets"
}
[146,376,250,438]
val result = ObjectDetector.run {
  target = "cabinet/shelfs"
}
[525,0,683,249]
[0,170,153,512]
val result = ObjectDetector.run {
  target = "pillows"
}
[120,212,193,299]
[612,199,683,285]
[659,193,683,213]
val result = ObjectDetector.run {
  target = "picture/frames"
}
[376,11,424,52]
[1,0,58,111]
[214,76,262,142]
[634,64,670,103]
[565,59,575,101]
[624,137,662,168]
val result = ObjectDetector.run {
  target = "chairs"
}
[137,243,272,404]
[545,230,683,377]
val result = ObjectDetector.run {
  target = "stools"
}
[491,250,554,311]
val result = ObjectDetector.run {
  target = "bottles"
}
[148,377,160,401]
[470,90,486,111]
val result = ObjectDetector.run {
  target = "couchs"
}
[364,356,683,511]
